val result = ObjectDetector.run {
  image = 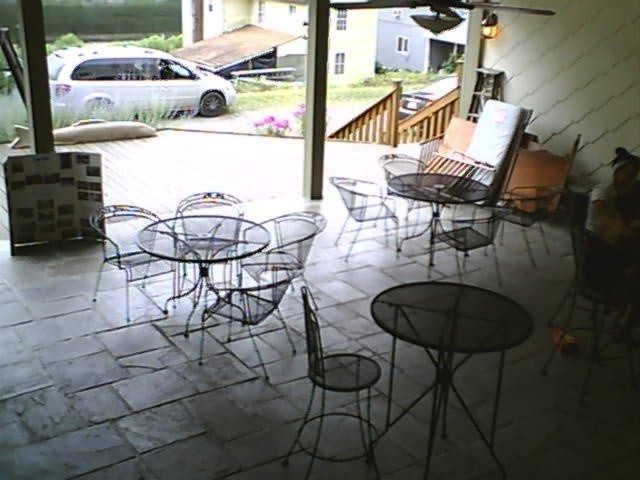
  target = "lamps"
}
[480,7,504,39]
[410,7,466,35]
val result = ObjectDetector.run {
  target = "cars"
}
[399,75,458,119]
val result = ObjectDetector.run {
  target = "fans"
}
[329,0,557,36]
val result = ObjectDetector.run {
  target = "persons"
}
[585,147,640,347]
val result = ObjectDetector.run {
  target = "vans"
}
[47,44,237,117]
